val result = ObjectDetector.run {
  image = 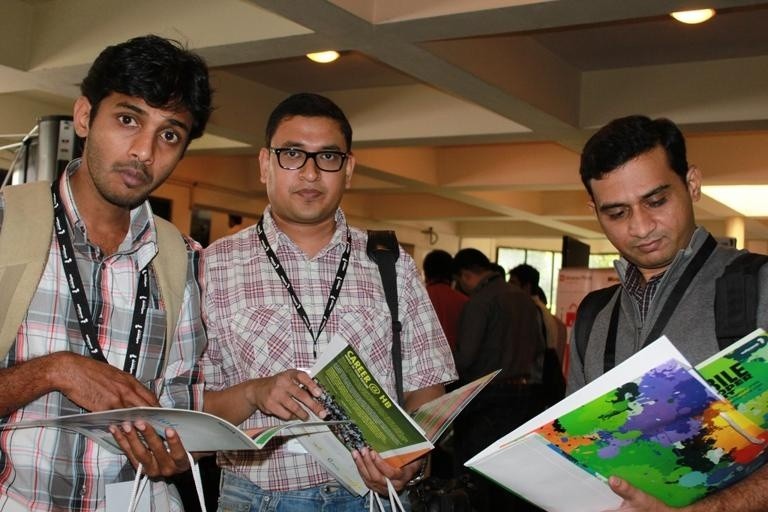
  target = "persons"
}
[421,247,568,510]
[559,113,768,511]
[200,92,459,512]
[1,33,218,512]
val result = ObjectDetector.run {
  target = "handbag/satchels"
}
[452,383,545,452]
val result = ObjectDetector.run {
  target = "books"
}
[280,332,503,498]
[1,406,350,458]
[462,327,768,510]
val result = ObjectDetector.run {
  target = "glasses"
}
[268,147,348,172]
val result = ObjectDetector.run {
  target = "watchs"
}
[406,456,429,488]
